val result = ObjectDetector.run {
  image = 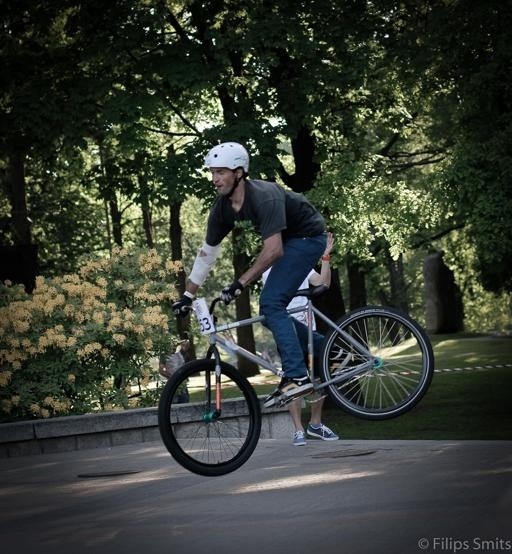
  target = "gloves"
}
[171,295,193,320]
[219,279,244,305]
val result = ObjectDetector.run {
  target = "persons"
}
[158,338,190,404]
[174,142,325,408]
[263,232,340,446]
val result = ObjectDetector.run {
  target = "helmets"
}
[202,142,250,174]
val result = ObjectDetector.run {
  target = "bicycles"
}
[158,278,435,476]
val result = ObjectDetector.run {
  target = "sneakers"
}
[291,431,308,446]
[307,421,339,441]
[263,374,314,408]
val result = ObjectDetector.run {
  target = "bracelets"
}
[183,290,195,299]
[320,256,331,261]
